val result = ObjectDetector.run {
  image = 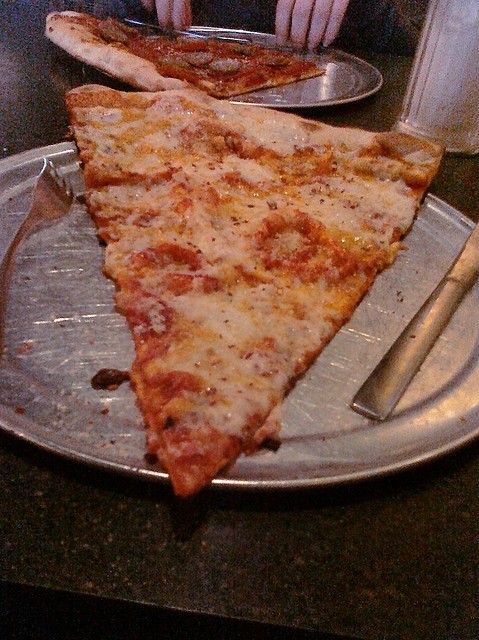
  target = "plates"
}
[86,24,384,108]
[2,140,478,490]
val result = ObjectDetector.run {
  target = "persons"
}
[138,3,358,51]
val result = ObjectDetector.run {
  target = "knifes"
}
[349,227,478,422]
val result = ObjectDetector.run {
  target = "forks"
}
[122,15,252,44]
[1,155,74,347]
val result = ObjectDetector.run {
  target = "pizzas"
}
[44,9,327,98]
[64,83,447,500]
